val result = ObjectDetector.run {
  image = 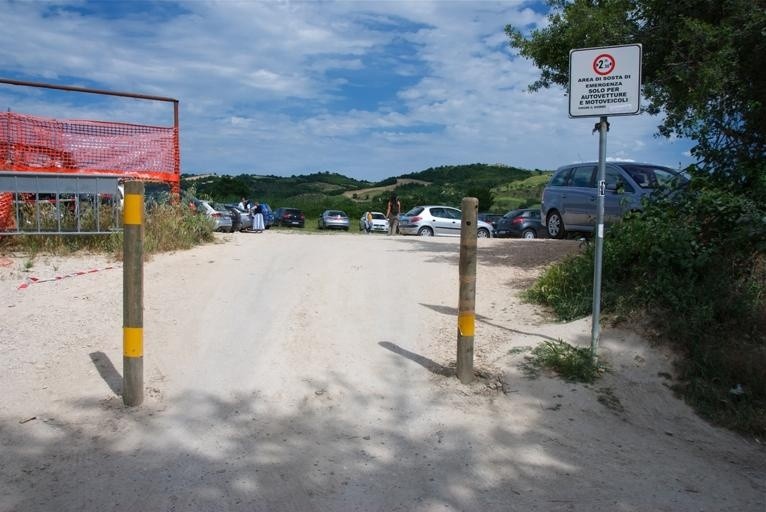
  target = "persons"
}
[366,210,374,233]
[251,202,265,233]
[386,192,401,236]
[238,197,250,212]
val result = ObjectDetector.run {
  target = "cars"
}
[0,139,78,171]
[399,205,494,239]
[359,212,390,234]
[273,207,305,228]
[12,187,274,232]
[478,212,504,229]
[395,212,407,233]
[493,209,548,239]
[318,209,349,231]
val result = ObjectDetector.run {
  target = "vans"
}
[540,162,691,239]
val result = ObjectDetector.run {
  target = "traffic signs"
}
[569,44,642,118]
